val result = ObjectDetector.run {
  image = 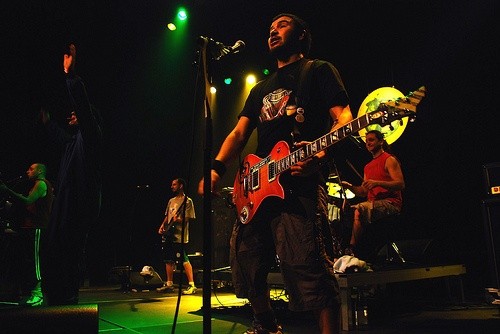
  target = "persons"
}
[199,14,354,334]
[156,178,198,295]
[39,42,101,305]
[0,162,53,307]
[349,131,405,245]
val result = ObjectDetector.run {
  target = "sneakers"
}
[182,286,198,295]
[157,284,175,293]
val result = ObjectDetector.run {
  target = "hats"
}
[270,12,311,57]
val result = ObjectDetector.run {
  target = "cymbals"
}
[325,181,356,200]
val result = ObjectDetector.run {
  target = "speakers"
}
[0,302,98,334]
[107,265,164,289]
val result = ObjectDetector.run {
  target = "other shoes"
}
[242,322,283,334]
[26,295,45,307]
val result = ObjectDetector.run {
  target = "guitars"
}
[231,85,427,224]
[158,193,191,248]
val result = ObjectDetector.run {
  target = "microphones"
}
[6,176,22,184]
[216,40,245,61]
[137,184,149,189]
